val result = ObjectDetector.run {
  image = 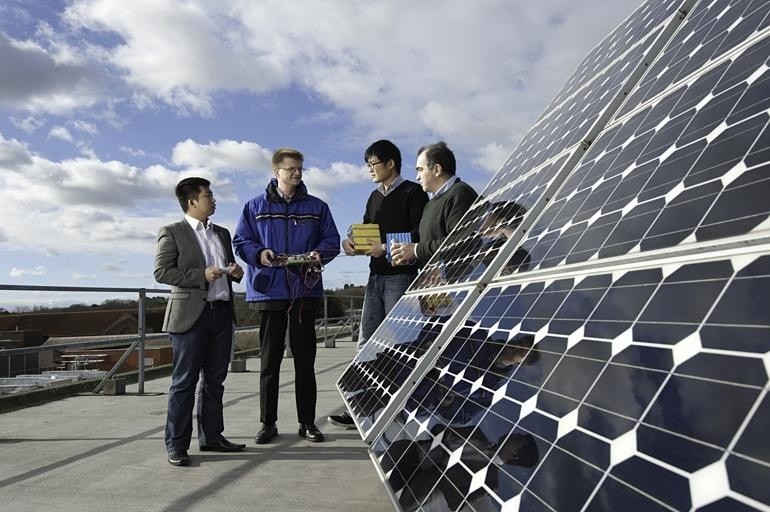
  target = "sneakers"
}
[326,411,356,428]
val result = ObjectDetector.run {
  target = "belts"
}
[203,299,231,311]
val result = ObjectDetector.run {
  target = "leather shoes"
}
[297,423,324,442]
[254,422,279,445]
[199,436,247,453]
[167,448,190,467]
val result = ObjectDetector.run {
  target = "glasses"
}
[366,161,382,169]
[279,167,306,174]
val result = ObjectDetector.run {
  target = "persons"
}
[328,139,429,429]
[231,151,339,444]
[153,176,246,466]
[337,314,541,426]
[411,198,532,317]
[379,424,536,512]
[390,140,477,267]
